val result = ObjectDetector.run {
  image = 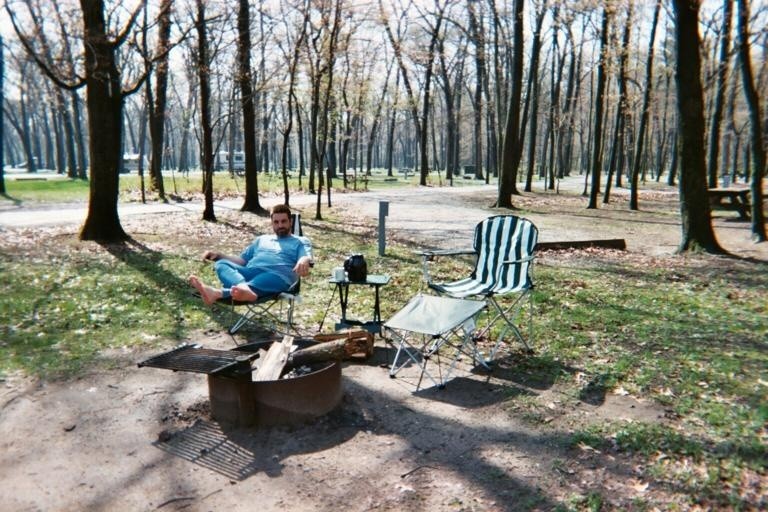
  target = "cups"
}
[333,266,345,281]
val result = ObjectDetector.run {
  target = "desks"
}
[706,187,752,223]
[328,274,393,326]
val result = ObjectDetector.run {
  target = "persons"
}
[185,202,312,306]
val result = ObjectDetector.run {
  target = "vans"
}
[209,150,247,171]
[118,153,150,172]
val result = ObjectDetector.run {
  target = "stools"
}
[383,291,494,390]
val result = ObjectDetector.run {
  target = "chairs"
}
[428,214,539,360]
[217,212,315,340]
